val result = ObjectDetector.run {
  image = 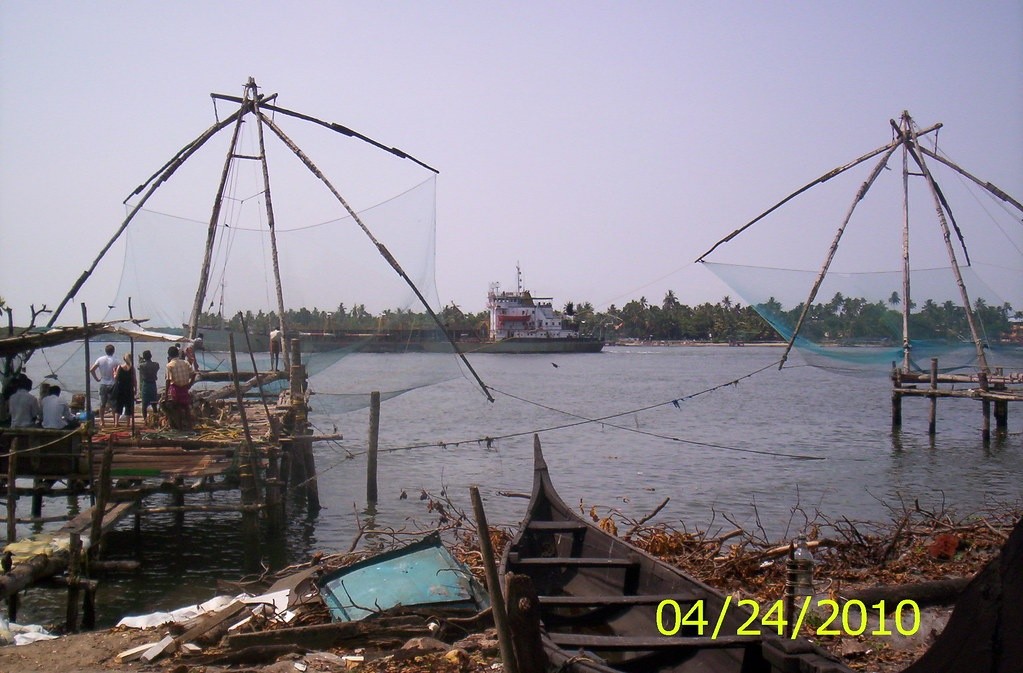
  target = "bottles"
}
[793,534,818,611]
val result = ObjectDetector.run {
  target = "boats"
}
[485,264,601,341]
[468,435,853,673]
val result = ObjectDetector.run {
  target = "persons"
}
[165,346,196,432]
[137,350,160,425]
[167,343,186,362]
[89,344,122,425]
[38,386,81,431]
[269,327,282,372]
[108,354,137,428]
[186,332,207,372]
[7,379,40,427]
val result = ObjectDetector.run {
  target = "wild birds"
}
[552,363,560,369]
